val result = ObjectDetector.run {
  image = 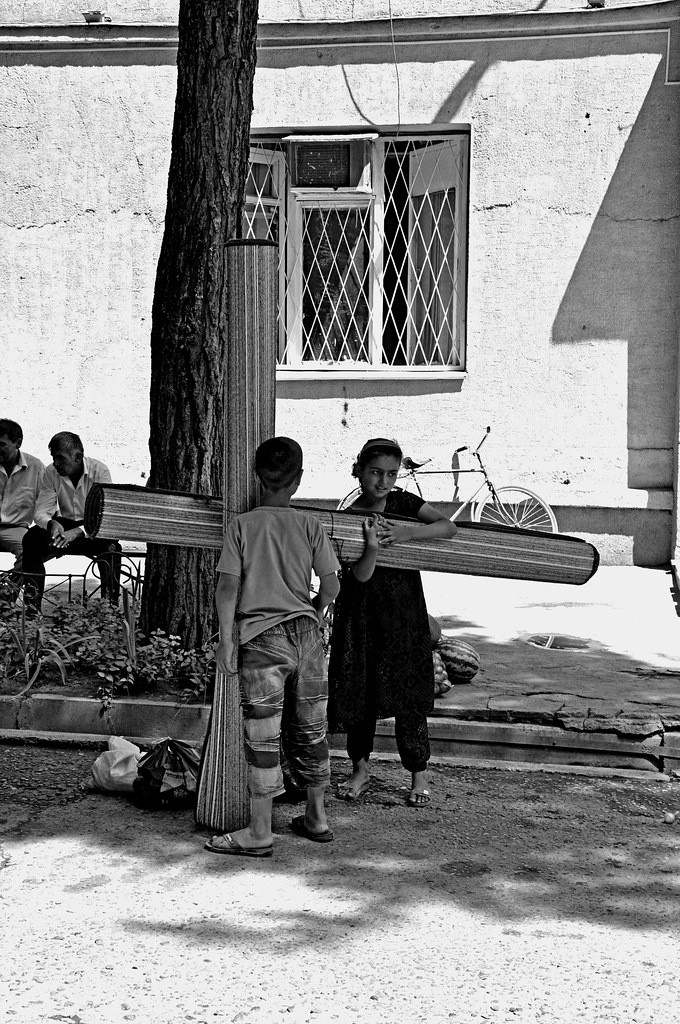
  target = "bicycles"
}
[337,426,559,534]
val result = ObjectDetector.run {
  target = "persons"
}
[204,435,340,857]
[0,418,49,614]
[22,432,123,618]
[326,437,458,807]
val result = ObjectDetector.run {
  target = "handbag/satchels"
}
[81,735,146,792]
[132,738,202,815]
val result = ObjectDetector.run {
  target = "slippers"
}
[408,784,432,806]
[205,832,273,857]
[287,814,335,843]
[336,778,370,802]
